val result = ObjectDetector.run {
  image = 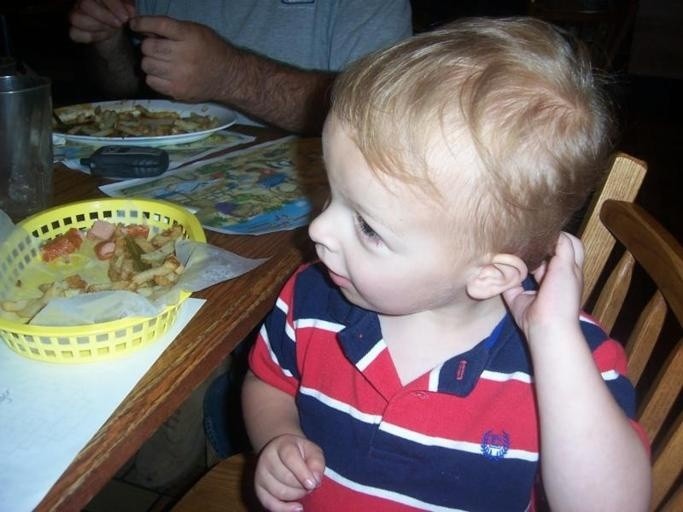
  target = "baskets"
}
[0,196,208,365]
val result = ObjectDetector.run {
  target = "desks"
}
[1,114,324,511]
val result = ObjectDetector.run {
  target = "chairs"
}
[172,153,681,512]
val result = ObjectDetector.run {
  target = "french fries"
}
[2,223,184,318]
[66,104,218,137]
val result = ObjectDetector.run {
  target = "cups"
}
[1,74,55,217]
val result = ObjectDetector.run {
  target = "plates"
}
[53,97,240,148]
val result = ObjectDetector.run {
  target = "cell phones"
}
[80,147,169,178]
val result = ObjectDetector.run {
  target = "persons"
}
[70,0,412,469]
[241,18,653,512]
